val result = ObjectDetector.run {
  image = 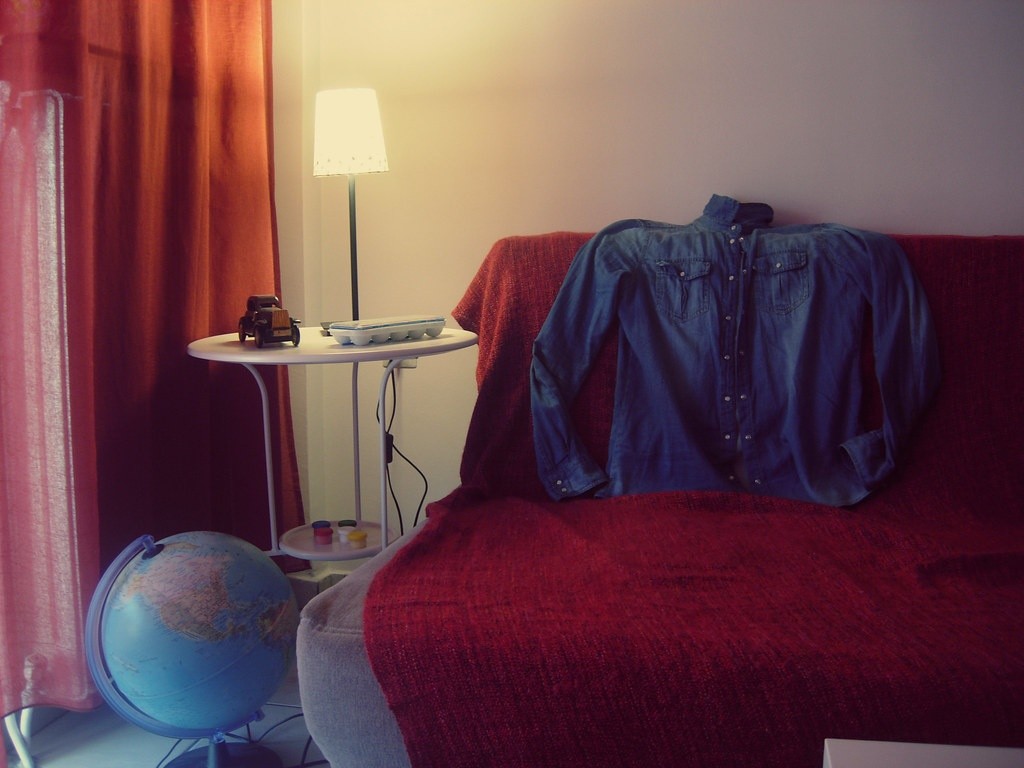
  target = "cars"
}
[239,296,302,349]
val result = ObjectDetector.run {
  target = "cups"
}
[313,519,334,547]
[337,519,369,549]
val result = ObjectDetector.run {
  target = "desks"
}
[188,328,478,557]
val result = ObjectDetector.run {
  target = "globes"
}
[83,529,301,768]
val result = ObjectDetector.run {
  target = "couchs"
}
[296,236,1024,768]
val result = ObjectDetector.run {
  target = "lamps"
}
[312,87,389,336]
